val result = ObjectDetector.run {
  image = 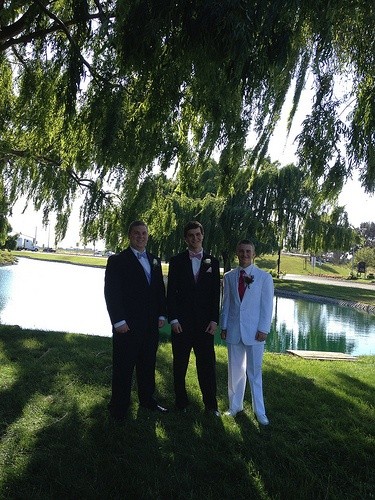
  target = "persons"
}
[222,239,274,426]
[104,220,166,422]
[166,221,221,419]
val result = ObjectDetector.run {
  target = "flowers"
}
[153,259,158,265]
[203,259,211,264]
[244,276,254,288]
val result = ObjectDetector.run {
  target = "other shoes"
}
[258,415,268,425]
[223,407,243,417]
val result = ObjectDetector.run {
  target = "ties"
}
[239,269,248,302]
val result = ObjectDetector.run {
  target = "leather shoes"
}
[175,400,189,412]
[139,401,168,413]
[205,404,220,417]
[111,396,130,407]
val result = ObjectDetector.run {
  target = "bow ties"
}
[190,254,202,258]
[136,252,148,257]
